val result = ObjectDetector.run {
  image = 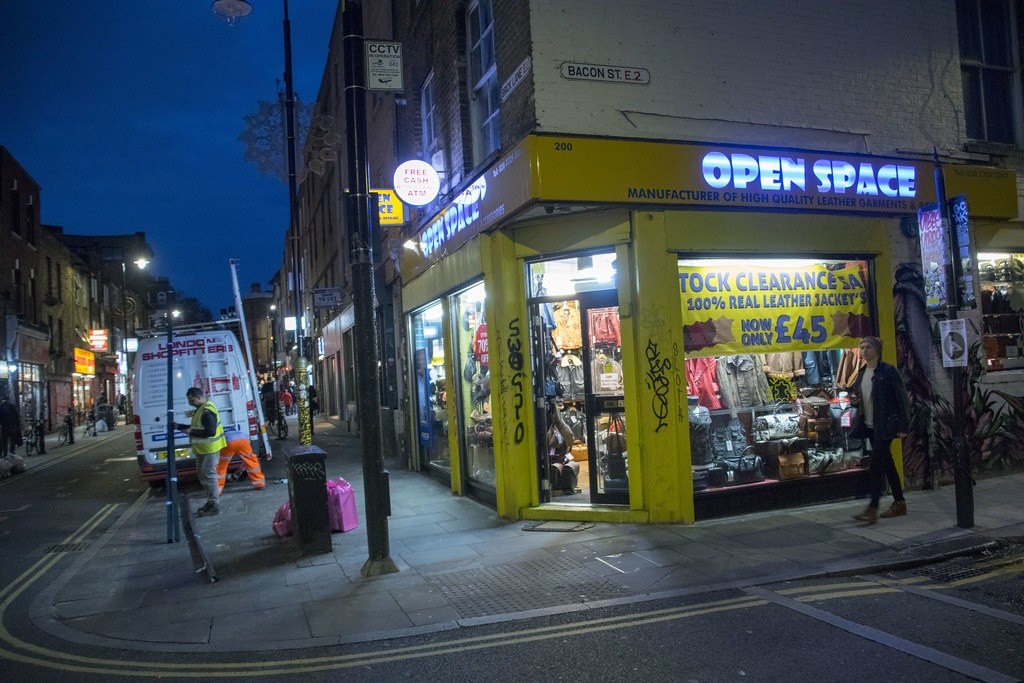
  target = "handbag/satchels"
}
[542,352,579,491]
[751,402,800,440]
[777,450,806,481]
[605,413,625,453]
[806,445,847,475]
[608,453,625,479]
[462,355,494,449]
[808,419,836,442]
[718,446,764,487]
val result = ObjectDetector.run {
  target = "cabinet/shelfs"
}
[979,280,1024,361]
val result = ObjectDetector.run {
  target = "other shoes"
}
[854,508,877,523]
[880,501,908,518]
[198,505,221,517]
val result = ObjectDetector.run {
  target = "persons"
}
[851,337,908,522]
[97,391,107,405]
[259,377,276,425]
[174,387,227,518]
[116,390,126,415]
[0,396,18,457]
[216,431,266,495]
[280,381,297,416]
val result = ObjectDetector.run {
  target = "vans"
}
[131,329,263,488]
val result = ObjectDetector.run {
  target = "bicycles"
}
[267,406,288,439]
[54,411,81,446]
[22,418,49,457]
[82,416,96,438]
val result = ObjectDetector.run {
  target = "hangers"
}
[561,300,571,317]
[567,406,578,412]
[596,350,607,361]
[566,352,575,365]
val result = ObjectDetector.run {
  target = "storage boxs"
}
[469,444,490,477]
[328,489,359,531]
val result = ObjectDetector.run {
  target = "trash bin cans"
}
[97,403,114,431]
[282,445,333,558]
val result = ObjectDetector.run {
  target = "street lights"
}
[119,256,152,422]
[209,0,315,446]
[267,305,282,440]
